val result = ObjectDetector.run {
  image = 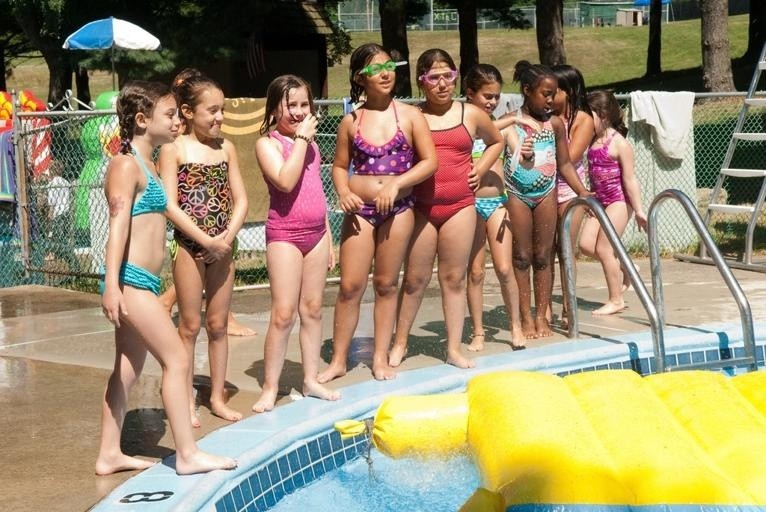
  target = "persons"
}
[317,41,438,381]
[154,65,255,337]
[497,57,596,340]
[249,76,342,413]
[539,62,595,331]
[160,76,243,426]
[94,79,241,477]
[576,86,650,319]
[457,63,542,353]
[387,46,505,371]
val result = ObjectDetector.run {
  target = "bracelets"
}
[294,135,310,145]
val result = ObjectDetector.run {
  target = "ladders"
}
[673,43,766,272]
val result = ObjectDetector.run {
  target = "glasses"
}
[358,61,396,76]
[419,70,458,85]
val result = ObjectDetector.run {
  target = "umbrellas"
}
[61,15,162,90]
